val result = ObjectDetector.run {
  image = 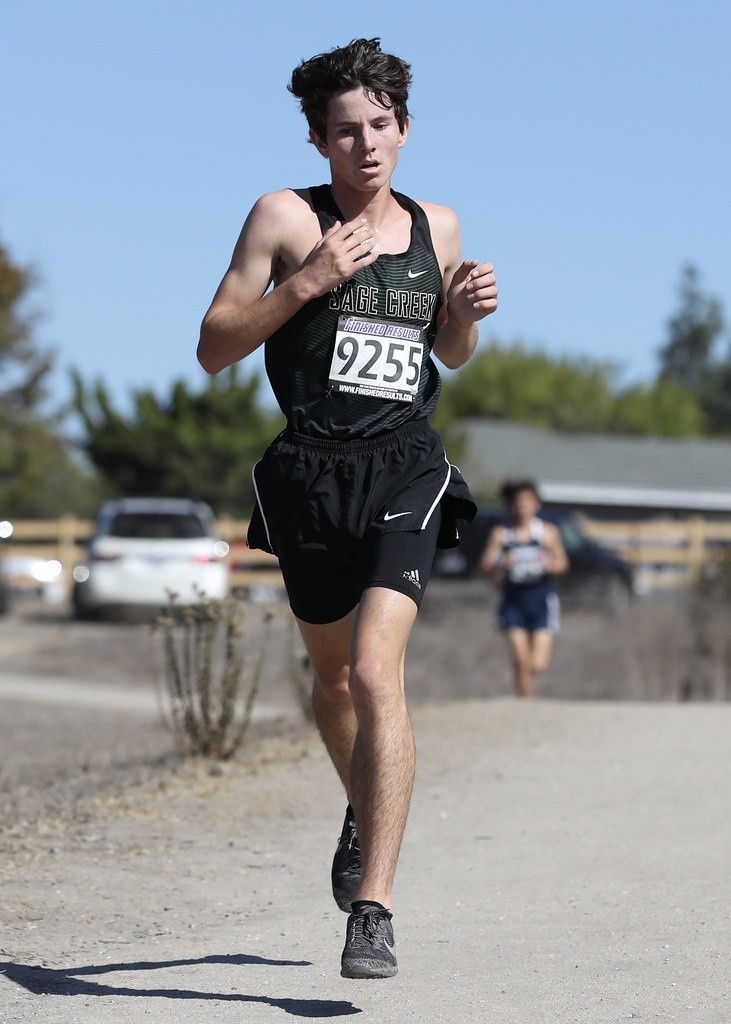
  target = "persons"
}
[197,36,497,980]
[482,480,570,699]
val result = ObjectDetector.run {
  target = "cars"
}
[72,499,229,618]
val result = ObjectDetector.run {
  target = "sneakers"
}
[340,900,399,980]
[331,803,362,913]
